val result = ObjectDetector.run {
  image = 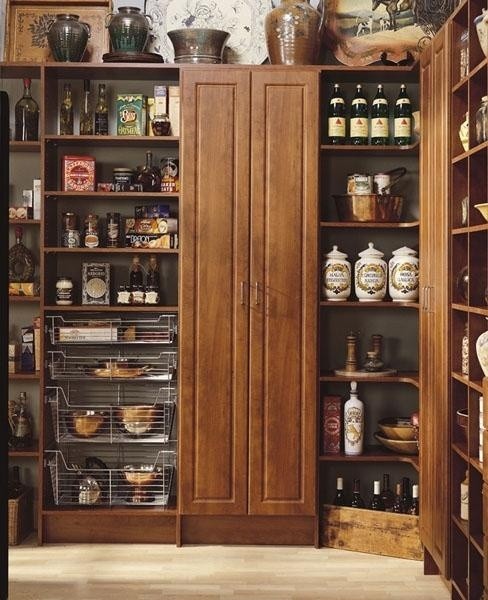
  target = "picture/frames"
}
[4,0,114,63]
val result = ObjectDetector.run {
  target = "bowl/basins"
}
[65,403,165,440]
[167,28,231,64]
[473,202,488,225]
[372,415,419,455]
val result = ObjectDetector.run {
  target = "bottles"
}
[333,473,420,516]
[8,225,35,284]
[112,150,179,192]
[15,78,108,141]
[60,211,121,249]
[9,392,35,448]
[461,321,469,376]
[326,83,413,144]
[344,381,366,456]
[8,465,26,499]
[129,255,160,306]
[459,469,470,521]
[318,242,420,301]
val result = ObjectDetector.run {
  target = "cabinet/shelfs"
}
[446,0,488,600]
[0,65,182,548]
[176,63,320,549]
[418,20,452,597]
[315,64,425,562]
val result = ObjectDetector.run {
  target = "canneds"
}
[55,276,73,305]
[62,211,76,228]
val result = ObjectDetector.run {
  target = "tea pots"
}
[71,457,111,505]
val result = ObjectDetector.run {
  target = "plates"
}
[102,51,165,63]
[144,0,274,64]
